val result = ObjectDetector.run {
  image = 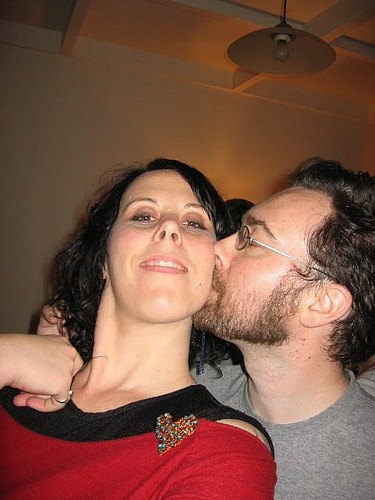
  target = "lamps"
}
[228,0,336,74]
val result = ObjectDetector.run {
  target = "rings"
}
[51,391,73,404]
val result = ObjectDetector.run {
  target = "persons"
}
[0,158,278,500]
[37,156,375,500]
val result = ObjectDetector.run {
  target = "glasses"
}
[235,220,355,310]
